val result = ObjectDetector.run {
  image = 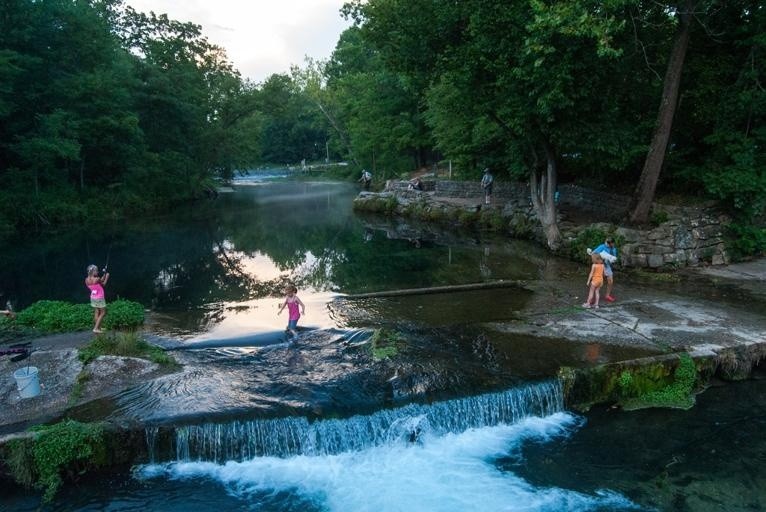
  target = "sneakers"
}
[582,302,593,309]
[604,294,615,301]
[591,303,601,311]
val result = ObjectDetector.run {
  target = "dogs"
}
[586,247,618,266]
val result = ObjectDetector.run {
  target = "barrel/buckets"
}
[13,366,41,399]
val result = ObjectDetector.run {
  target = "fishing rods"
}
[102,206,121,273]
[26,311,34,375]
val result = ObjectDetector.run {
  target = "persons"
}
[592,236,619,302]
[359,169,373,191]
[554,187,562,209]
[408,177,424,190]
[85,264,109,334]
[481,168,494,205]
[278,286,306,336]
[582,253,606,310]
